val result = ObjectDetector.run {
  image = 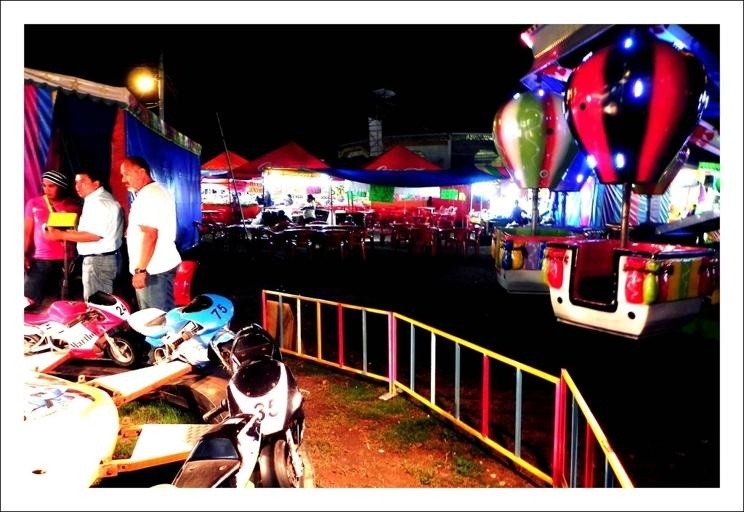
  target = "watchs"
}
[135,267,146,274]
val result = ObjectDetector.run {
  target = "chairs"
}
[202,206,485,263]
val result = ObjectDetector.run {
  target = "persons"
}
[426,196,432,206]
[300,194,315,223]
[511,200,521,218]
[23,170,83,298]
[287,194,292,205]
[120,156,182,311]
[230,197,242,222]
[41,171,125,304]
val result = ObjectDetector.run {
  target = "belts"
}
[83,250,118,256]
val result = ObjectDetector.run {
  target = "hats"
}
[42,170,70,190]
